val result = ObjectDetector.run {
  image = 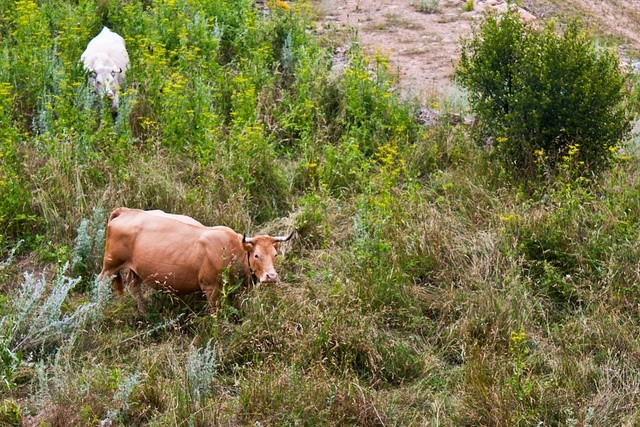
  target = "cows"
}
[98,206,294,313]
[81,26,130,121]
